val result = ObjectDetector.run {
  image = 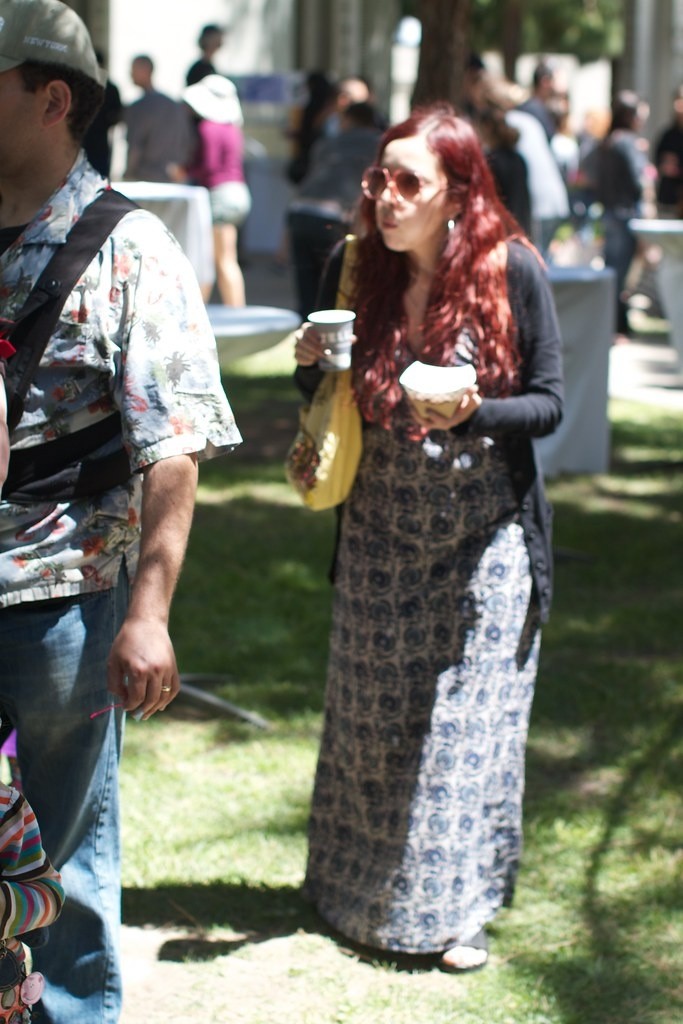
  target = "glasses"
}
[361,166,451,199]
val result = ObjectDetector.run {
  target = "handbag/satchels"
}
[285,232,362,512]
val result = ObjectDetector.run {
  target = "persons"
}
[292,100,566,972]
[288,47,683,321]
[0,0,255,1024]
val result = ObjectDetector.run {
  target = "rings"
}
[161,686,171,691]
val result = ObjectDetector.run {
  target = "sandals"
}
[440,931,490,973]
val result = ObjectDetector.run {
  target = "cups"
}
[307,309,356,373]
[399,360,477,422]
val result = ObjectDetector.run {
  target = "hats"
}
[182,74,242,124]
[1,1,108,89]
